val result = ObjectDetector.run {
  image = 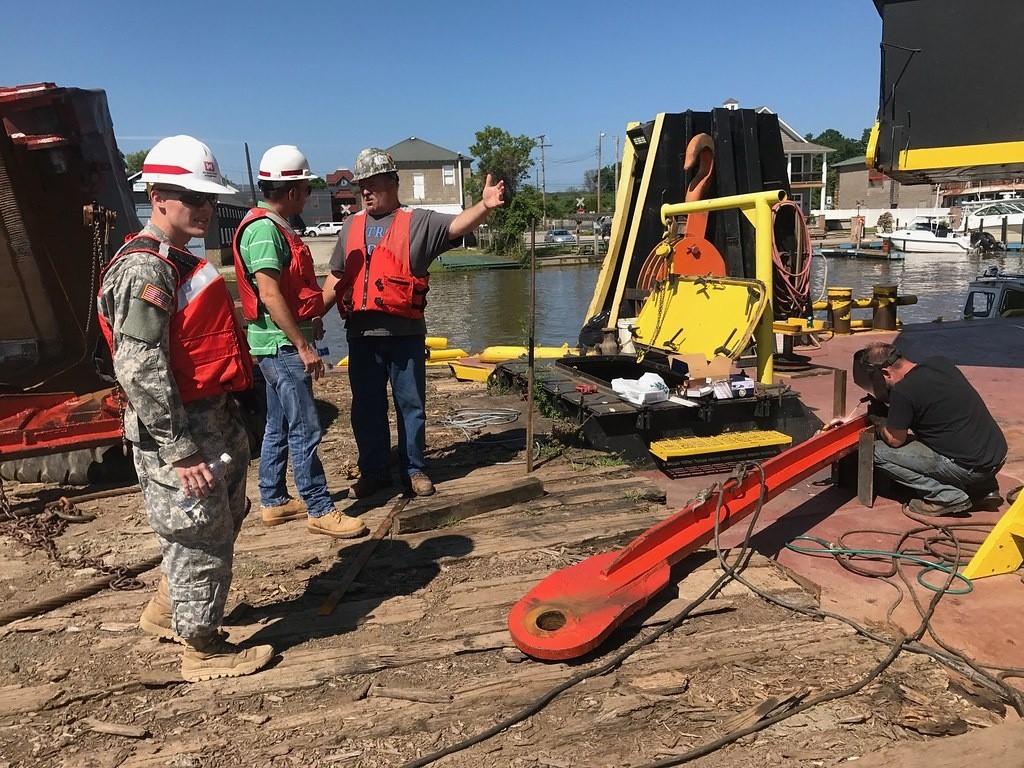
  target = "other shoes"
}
[909,495,972,516]
[347,475,393,499]
[967,486,1003,511]
[401,471,434,495]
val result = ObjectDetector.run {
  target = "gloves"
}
[867,401,889,434]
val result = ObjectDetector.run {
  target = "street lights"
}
[540,159,546,230]
[598,132,606,213]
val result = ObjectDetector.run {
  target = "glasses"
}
[288,185,312,196]
[155,188,219,208]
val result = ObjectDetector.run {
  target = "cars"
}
[593,215,613,239]
[292,227,303,237]
[543,230,577,249]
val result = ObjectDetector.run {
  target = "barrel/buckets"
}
[617,318,639,353]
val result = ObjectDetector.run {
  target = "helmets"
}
[133,135,240,194]
[350,148,398,183]
[257,145,320,180]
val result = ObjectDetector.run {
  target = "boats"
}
[873,177,985,255]
[946,181,1024,233]
[960,265,1024,322]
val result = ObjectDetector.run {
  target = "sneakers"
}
[306,507,367,538]
[138,575,224,645]
[180,631,274,683]
[261,495,307,526]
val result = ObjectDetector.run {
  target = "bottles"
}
[170,452,232,512]
[601,327,618,355]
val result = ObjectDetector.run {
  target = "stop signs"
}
[577,209,585,215]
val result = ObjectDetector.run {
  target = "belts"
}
[953,459,1007,473]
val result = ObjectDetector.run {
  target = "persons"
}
[321,148,506,499]
[853,342,1009,517]
[98,134,275,682]
[234,144,367,538]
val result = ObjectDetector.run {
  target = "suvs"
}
[304,222,344,238]
